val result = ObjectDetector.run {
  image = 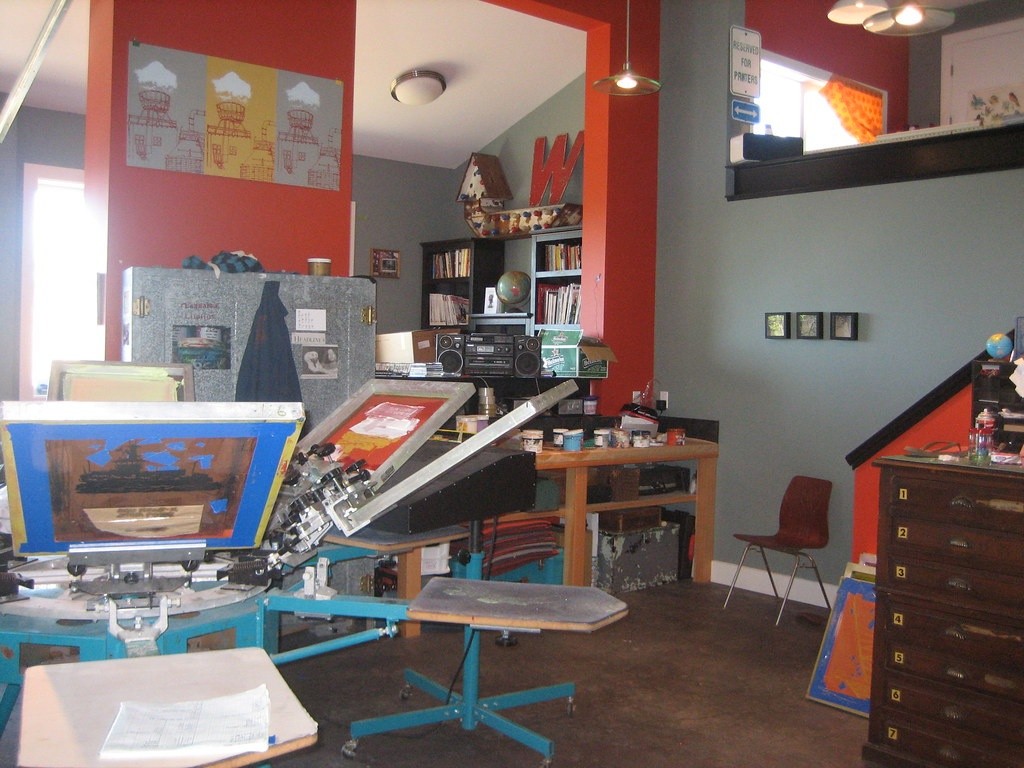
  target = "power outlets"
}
[659,391,669,409]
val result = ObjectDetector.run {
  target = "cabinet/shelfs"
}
[861,451,1024,768]
[420,225,583,337]
[121,266,376,428]
[536,434,718,596]
[971,359,1024,453]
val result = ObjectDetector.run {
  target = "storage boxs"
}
[596,521,681,596]
[484,287,505,314]
[536,329,618,379]
[558,397,582,414]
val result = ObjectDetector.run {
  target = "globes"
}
[985,333,1013,362]
[495,270,531,313]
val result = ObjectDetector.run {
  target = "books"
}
[545,243,581,270]
[538,283,580,325]
[432,248,470,279]
[429,294,468,325]
[1004,424,1024,432]
[409,362,442,377]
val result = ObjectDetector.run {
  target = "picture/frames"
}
[370,250,401,278]
[765,312,791,339]
[844,551,879,585]
[830,312,858,340]
[796,312,822,340]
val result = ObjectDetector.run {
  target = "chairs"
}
[724,475,832,626]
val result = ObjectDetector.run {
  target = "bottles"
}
[969,428,993,467]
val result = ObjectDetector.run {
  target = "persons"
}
[307,347,337,374]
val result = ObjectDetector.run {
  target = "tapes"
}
[477,388,497,417]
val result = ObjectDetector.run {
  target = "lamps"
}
[593,0,661,96]
[390,70,446,106]
[828,0,956,38]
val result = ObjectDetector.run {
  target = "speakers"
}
[514,335,542,378]
[435,333,466,376]
